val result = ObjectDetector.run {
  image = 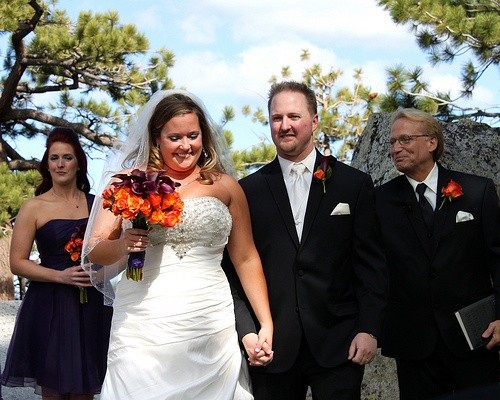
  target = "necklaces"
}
[174,178,198,192]
[53,187,79,208]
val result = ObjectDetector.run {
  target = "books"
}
[455,293,500,350]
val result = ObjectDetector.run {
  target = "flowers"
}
[65,226,94,302]
[439,180,463,210]
[311,160,332,192]
[101,168,184,281]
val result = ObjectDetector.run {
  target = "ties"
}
[290,164,309,245]
[416,183,434,229]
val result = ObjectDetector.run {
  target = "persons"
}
[0,124,114,400]
[373,108,500,400]
[80,88,274,400]
[221,81,391,400]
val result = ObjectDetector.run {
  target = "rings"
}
[366,359,369,362]
[131,241,136,248]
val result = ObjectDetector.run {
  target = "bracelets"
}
[370,333,378,339]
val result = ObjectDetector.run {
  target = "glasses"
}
[390,134,432,145]
